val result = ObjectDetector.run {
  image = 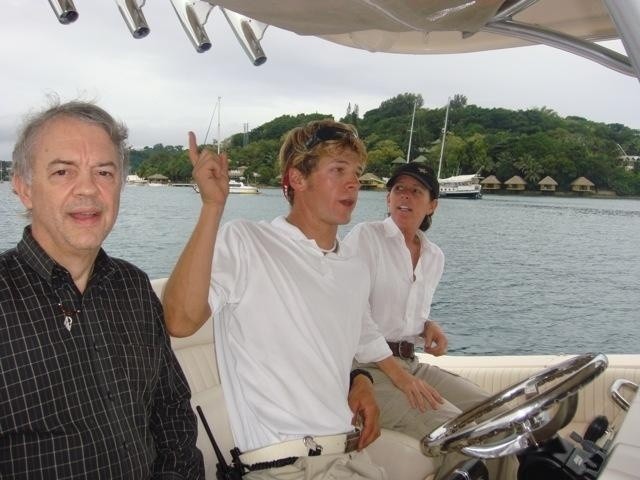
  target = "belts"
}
[386,342,415,360]
[236,429,361,473]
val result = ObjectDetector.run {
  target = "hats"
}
[386,164,438,199]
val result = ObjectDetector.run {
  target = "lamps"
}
[46,1,271,68]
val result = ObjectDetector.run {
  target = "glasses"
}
[300,126,355,152]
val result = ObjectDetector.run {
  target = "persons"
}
[0,88,205,480]
[340,162,520,480]
[161,119,434,480]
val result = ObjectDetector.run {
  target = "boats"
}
[371,96,485,199]
[192,95,262,194]
[127,173,170,187]
